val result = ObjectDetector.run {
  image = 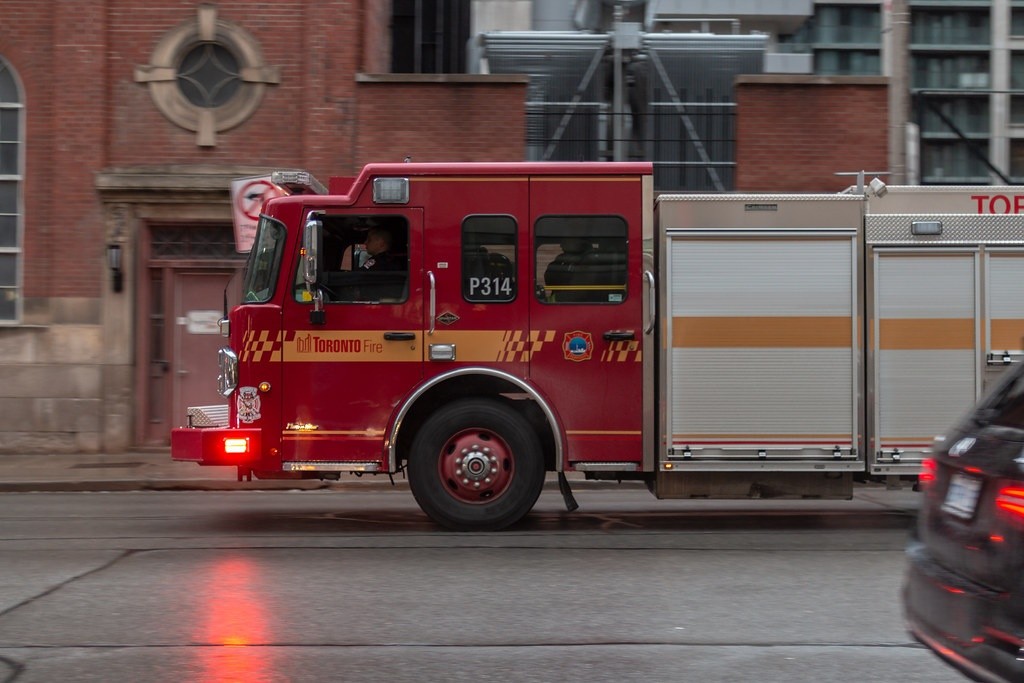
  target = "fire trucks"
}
[168,153,1023,531]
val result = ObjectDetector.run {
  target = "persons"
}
[314,223,404,301]
[544,238,614,302]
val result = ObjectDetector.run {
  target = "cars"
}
[896,361,1024,683]
